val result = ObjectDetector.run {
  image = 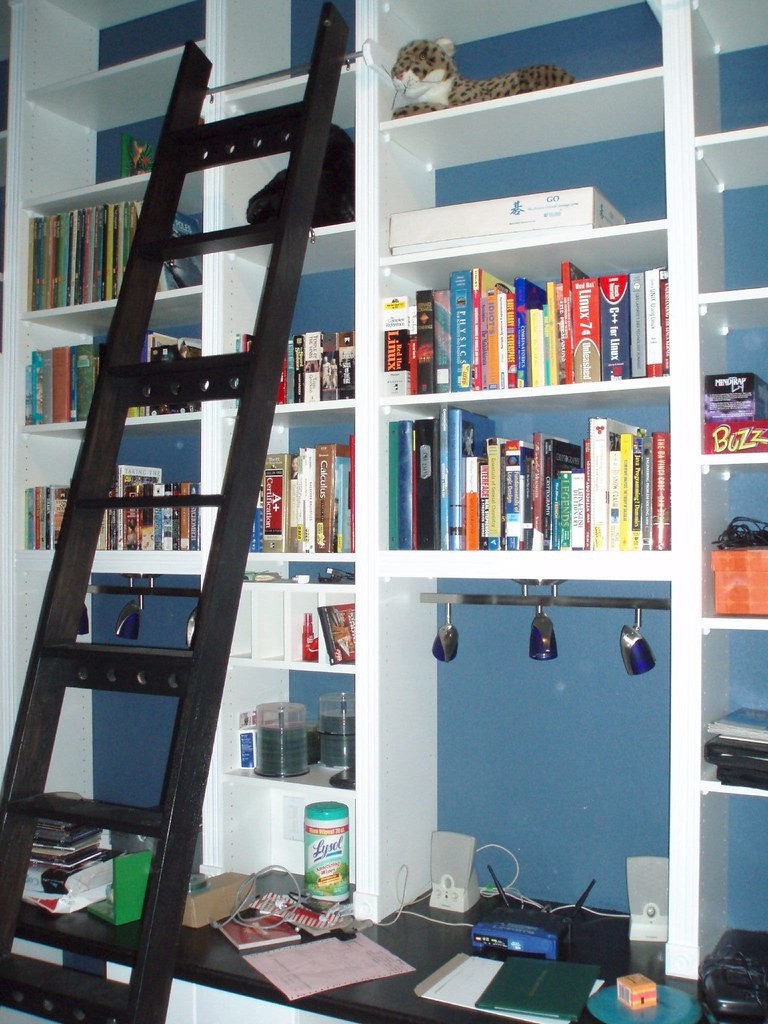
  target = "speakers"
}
[623,855,671,942]
[428,831,482,912]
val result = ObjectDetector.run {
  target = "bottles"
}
[304,800,350,902]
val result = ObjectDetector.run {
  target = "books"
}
[476,955,603,1022]
[317,603,356,666]
[698,704,767,791]
[16,184,678,555]
[21,813,114,869]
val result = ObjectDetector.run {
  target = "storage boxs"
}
[388,185,624,256]
[708,548,768,616]
[705,419,768,454]
[183,872,256,928]
[704,372,768,421]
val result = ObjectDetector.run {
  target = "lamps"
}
[76,568,203,649]
[422,581,670,680]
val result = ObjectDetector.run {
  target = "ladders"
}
[0,0,355,1024]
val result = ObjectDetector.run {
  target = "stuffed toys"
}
[389,37,577,118]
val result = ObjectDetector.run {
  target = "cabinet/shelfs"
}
[0,0,768,1024]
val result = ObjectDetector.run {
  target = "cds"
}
[254,708,357,777]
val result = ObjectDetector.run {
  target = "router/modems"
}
[470,865,597,959]
